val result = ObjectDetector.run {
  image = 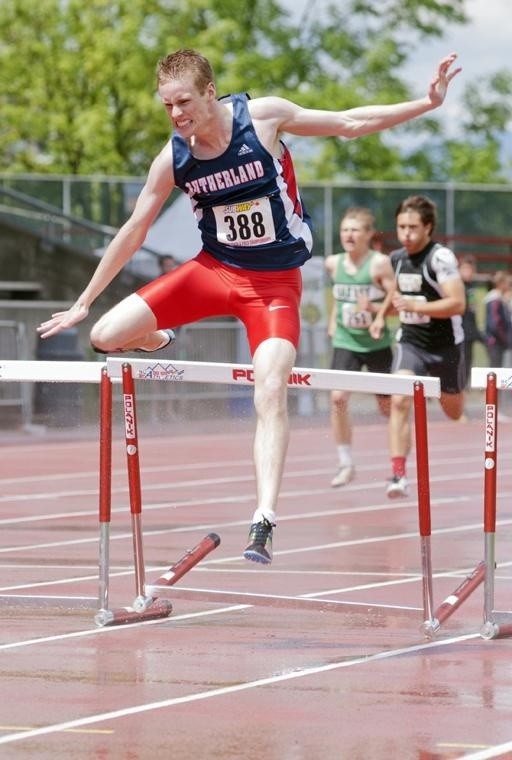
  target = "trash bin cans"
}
[32,327,85,426]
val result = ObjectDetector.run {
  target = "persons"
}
[455,255,484,406]
[485,268,511,368]
[385,194,468,498]
[323,207,396,488]
[36,50,463,564]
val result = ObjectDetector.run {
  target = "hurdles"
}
[470,366,512,640]
[0,358,176,628]
[105,355,496,638]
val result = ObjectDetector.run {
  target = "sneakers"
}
[331,464,354,486]
[90,329,175,354]
[387,477,407,498]
[243,523,272,563]
